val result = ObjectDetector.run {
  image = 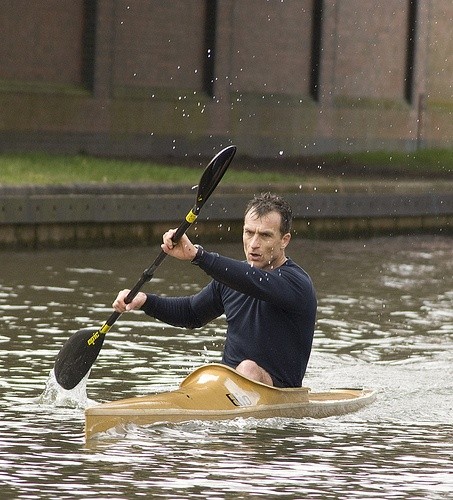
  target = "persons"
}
[113,196,316,388]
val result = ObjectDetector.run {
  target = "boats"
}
[83,363,379,450]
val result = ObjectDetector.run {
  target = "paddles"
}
[54,145,238,390]
